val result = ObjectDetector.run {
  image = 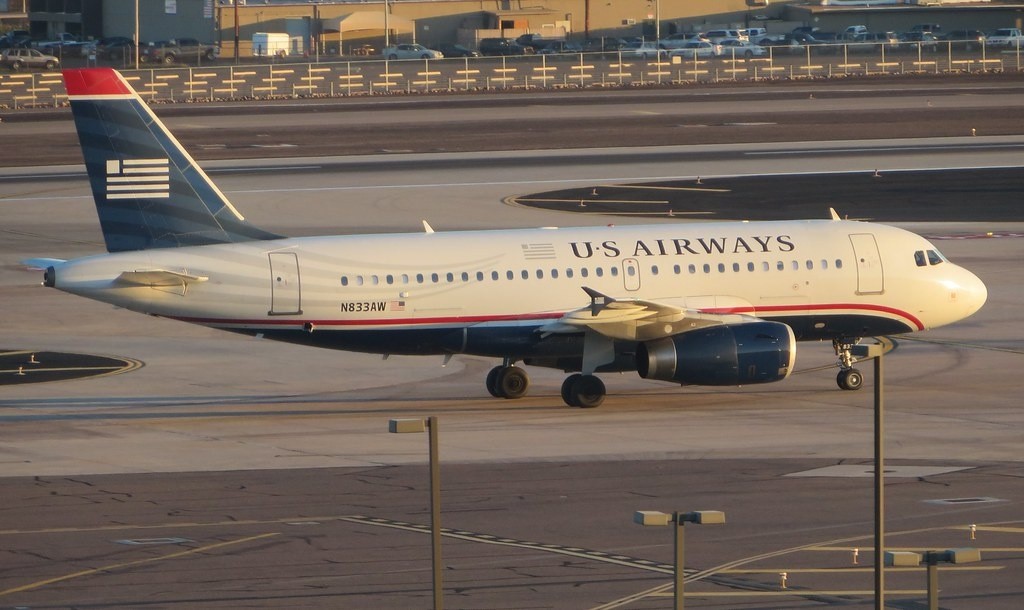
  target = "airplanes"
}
[13,68,988,409]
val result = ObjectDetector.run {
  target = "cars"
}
[381,43,444,63]
[516,23,945,57]
[939,30,985,53]
[1,47,60,73]
[0,29,220,65]
[431,42,480,61]
[984,27,1024,51]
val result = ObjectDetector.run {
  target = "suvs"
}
[479,37,534,56]
